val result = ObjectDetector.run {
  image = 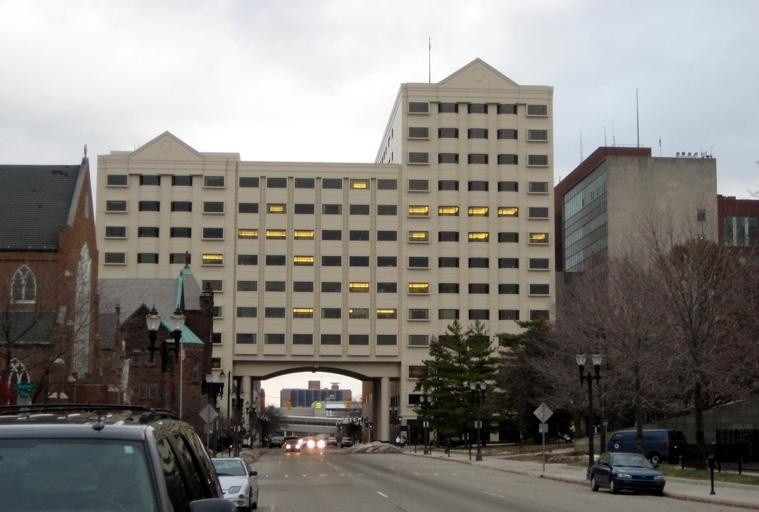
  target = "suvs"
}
[0,402,236,512]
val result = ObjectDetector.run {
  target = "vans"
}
[607,429,687,464]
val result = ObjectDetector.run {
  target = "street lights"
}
[206,366,225,459]
[420,396,432,454]
[469,380,486,461]
[575,348,603,466]
[146,303,186,419]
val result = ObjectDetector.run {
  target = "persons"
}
[443,435,449,453]
[395,434,409,448]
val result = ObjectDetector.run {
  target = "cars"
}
[209,456,258,512]
[269,437,337,452]
[589,452,665,496]
[341,437,353,448]
